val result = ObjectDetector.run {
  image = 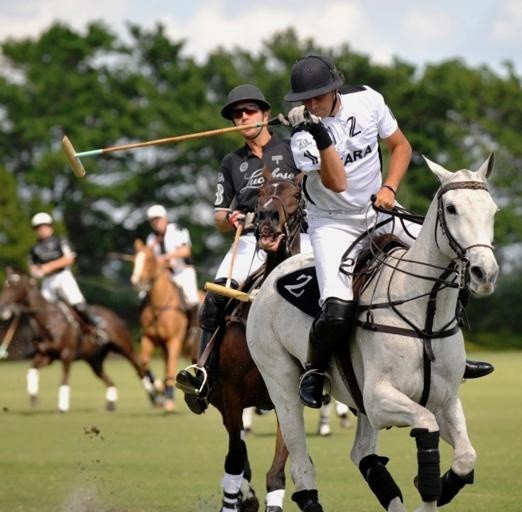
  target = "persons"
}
[175,83,313,415]
[3,265,36,358]
[25,211,106,345]
[281,52,496,411]
[136,204,200,329]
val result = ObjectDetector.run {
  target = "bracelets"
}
[383,184,397,196]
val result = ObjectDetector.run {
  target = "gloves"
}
[277,105,332,149]
[226,211,246,231]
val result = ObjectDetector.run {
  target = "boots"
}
[176,278,239,414]
[463,359,494,378]
[299,297,357,408]
[73,302,102,326]
[184,303,198,346]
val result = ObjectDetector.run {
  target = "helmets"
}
[31,212,52,226]
[147,205,166,221]
[220,84,271,120]
[284,54,344,102]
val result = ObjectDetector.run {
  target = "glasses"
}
[231,104,260,118]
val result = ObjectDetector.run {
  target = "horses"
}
[191,164,308,512]
[0,264,165,413]
[246,151,500,512]
[131,238,206,411]
